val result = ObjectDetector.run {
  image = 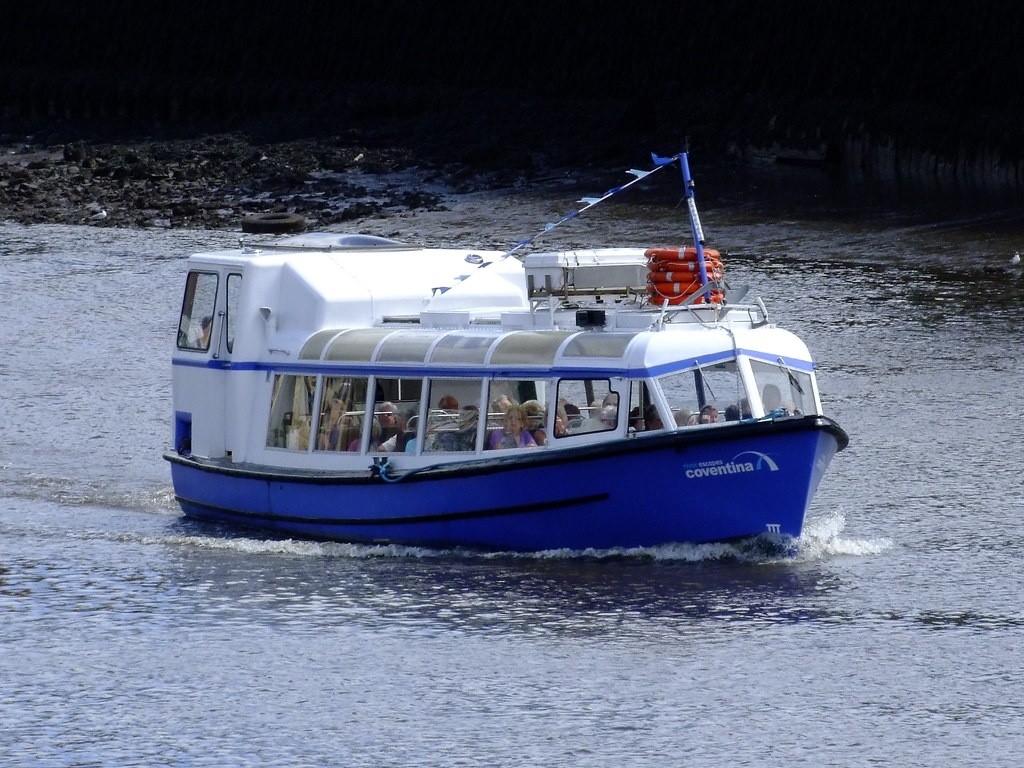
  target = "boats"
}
[162,144,853,549]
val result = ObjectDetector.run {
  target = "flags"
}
[478,262,493,268]
[651,152,673,166]
[432,286,452,296]
[625,169,650,178]
[577,198,602,205]
[454,274,470,282]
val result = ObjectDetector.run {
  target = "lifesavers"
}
[645,246,723,304]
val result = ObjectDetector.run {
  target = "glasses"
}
[606,403,617,406]
[508,416,521,421]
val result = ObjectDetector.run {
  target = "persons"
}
[674,384,782,427]
[316,391,663,452]
[187,317,212,349]
